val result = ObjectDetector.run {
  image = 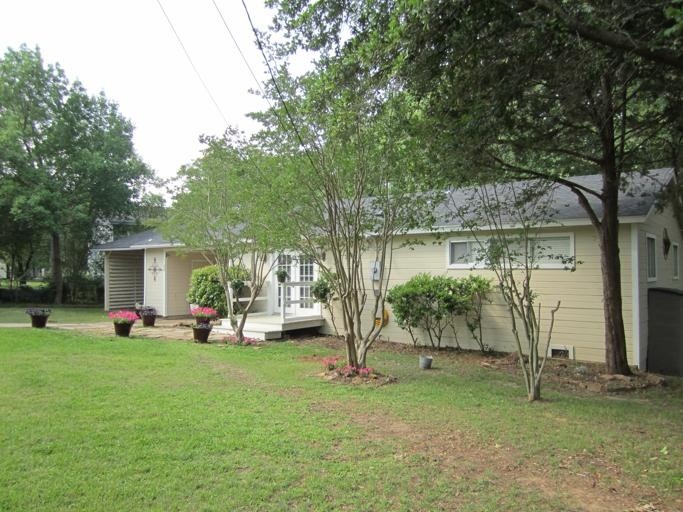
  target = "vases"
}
[143,315,156,326]
[115,321,134,336]
[32,314,49,327]
[192,327,211,343]
[196,316,210,325]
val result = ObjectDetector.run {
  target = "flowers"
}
[108,310,141,323]
[192,306,217,319]
[135,306,157,316]
[26,307,53,317]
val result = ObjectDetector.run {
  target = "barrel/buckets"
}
[419,349,433,369]
